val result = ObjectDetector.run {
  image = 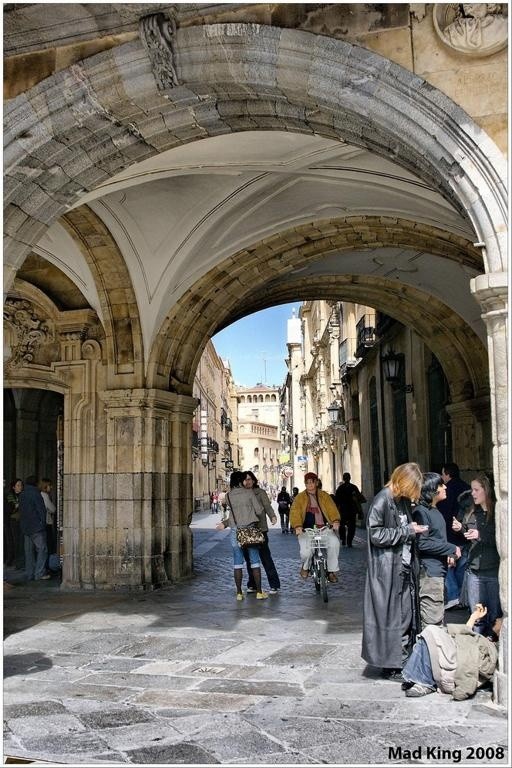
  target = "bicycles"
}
[296,522,338,603]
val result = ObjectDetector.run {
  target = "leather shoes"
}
[387,669,408,682]
[326,572,339,583]
[301,562,310,578]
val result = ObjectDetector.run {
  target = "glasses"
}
[305,479,315,484]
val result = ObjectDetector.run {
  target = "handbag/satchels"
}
[236,527,266,549]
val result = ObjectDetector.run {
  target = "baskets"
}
[306,534,329,549]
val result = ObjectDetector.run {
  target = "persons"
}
[451,472,497,635]
[7,479,25,565]
[288,487,303,534]
[40,477,59,576]
[400,604,501,700]
[410,473,463,626]
[223,471,269,600]
[335,471,362,548]
[436,462,472,611]
[20,476,52,580]
[277,486,289,535]
[361,462,430,681]
[287,472,343,583]
[211,488,226,514]
[215,472,283,595]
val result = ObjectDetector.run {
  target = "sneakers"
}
[268,588,277,594]
[4,580,17,593]
[246,587,256,593]
[256,590,269,600]
[405,684,437,698]
[444,599,459,610]
[237,592,246,601]
[35,574,51,581]
